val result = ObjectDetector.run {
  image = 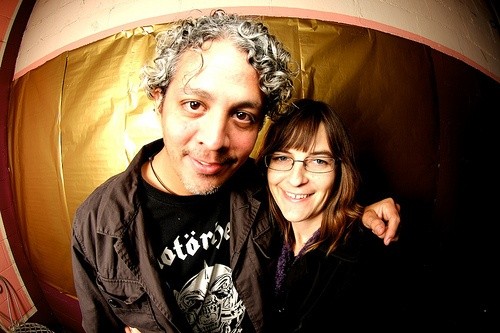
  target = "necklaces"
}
[147,153,179,196]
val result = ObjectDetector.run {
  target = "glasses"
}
[263,153,338,172]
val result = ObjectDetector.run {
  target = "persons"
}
[254,98,424,333]
[71,8,401,333]
